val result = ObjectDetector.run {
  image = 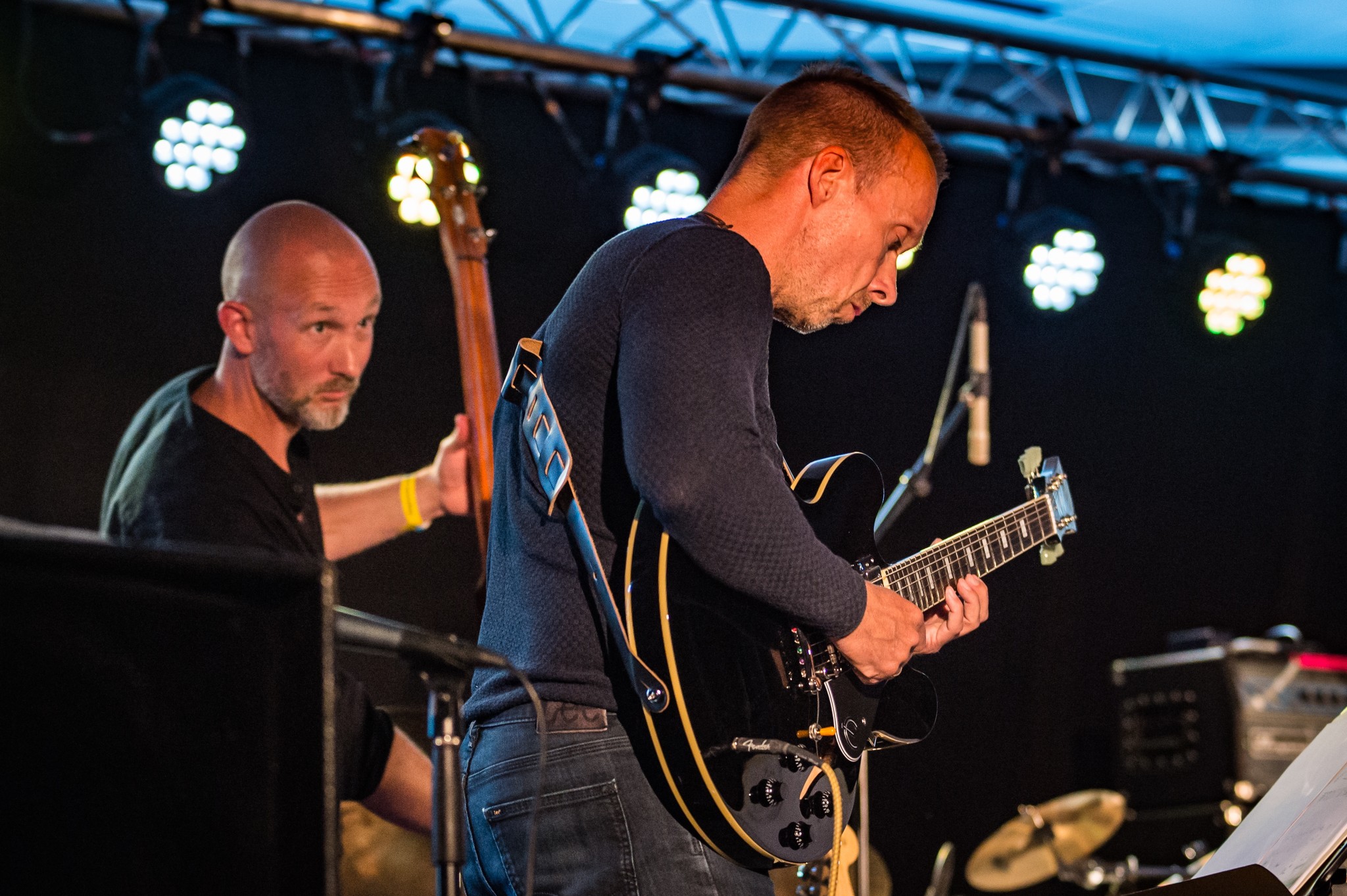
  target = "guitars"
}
[617,445,1082,876]
[392,125,506,601]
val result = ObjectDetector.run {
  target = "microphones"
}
[970,296,990,466]
[333,604,509,671]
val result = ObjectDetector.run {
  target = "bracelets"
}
[400,472,433,533]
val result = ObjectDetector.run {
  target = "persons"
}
[458,64,991,896]
[100,199,473,896]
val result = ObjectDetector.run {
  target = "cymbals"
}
[964,788,1128,892]
[924,841,956,896]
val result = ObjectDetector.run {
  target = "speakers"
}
[1,519,334,896]
[1111,643,1347,896]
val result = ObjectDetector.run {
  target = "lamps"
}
[994,132,1109,321]
[1148,163,1281,344]
[130,1,258,200]
[369,30,489,244]
[553,67,710,235]
